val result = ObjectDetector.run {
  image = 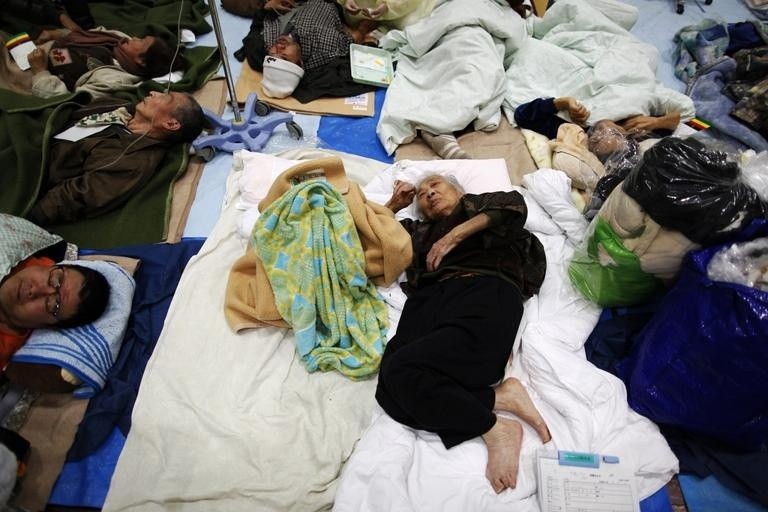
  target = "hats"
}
[260,54,305,99]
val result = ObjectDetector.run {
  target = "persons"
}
[248,1,682,164]
[0,241,112,375]
[387,169,552,494]
[27,26,176,105]
[0,0,89,30]
[29,91,204,225]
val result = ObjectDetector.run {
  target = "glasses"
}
[45,264,65,318]
